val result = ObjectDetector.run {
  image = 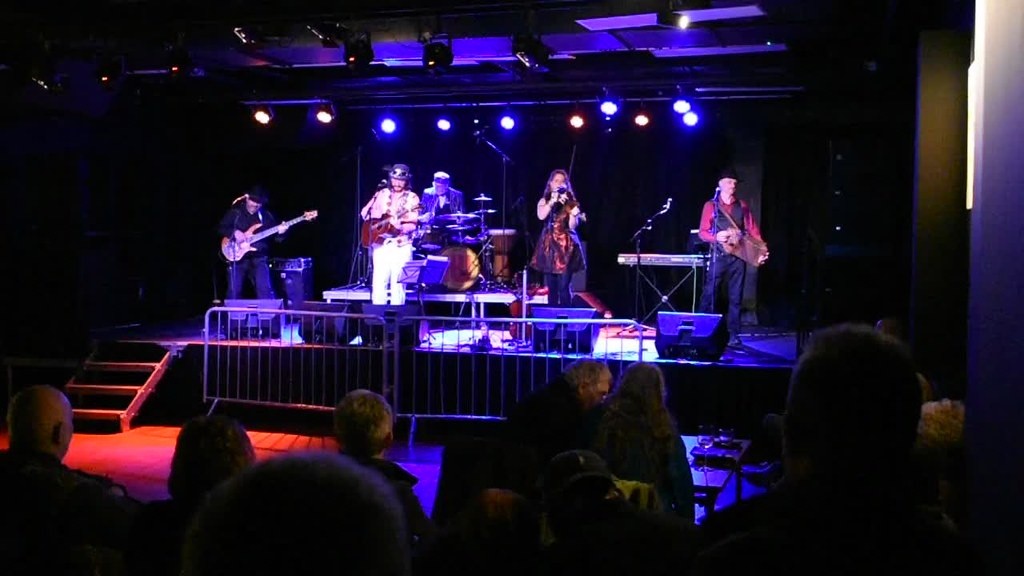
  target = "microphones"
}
[715,187,720,200]
[377,178,387,188]
[232,193,251,205]
[371,129,381,141]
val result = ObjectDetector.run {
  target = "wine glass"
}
[697,425,714,471]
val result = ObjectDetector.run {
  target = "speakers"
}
[529,304,601,354]
[359,302,420,344]
[299,301,353,343]
[224,298,286,336]
[655,311,730,359]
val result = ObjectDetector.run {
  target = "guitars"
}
[360,202,423,247]
[221,210,318,263]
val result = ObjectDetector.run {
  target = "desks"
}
[681,436,752,526]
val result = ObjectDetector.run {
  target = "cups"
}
[719,428,734,448]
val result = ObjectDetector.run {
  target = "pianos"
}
[617,253,705,336]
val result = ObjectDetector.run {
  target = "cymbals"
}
[437,214,481,220]
[471,208,496,213]
[474,194,493,201]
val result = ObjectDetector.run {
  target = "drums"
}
[440,247,480,292]
[489,228,516,283]
[451,225,487,246]
[417,225,445,251]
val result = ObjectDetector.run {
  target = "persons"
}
[361,164,420,306]
[529,170,589,307]
[0,321,970,576]
[697,171,770,348]
[420,172,466,232]
[219,186,290,299]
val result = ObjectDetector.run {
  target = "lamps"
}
[307,16,376,66]
[655,11,689,30]
[514,33,549,69]
[422,31,455,68]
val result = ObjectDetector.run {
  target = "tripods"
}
[473,201,510,294]
[611,210,663,334]
[330,137,372,290]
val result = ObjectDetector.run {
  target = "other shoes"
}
[730,332,741,345]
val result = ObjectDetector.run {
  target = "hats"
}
[545,450,611,495]
[389,164,412,180]
[243,187,269,203]
[716,168,740,184]
[434,172,449,184]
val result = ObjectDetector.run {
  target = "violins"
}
[557,189,587,223]
